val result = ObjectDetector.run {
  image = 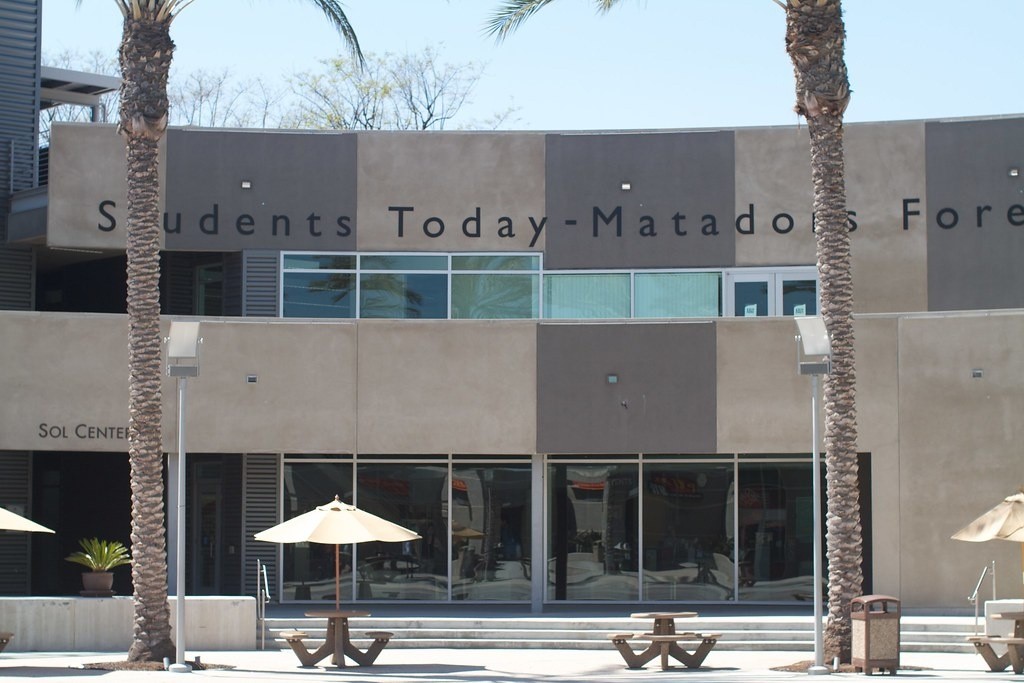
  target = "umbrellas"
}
[0,508,56,533]
[253,494,422,610]
[455,529,485,545]
[950,493,1024,542]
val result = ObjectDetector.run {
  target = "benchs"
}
[277,630,311,667]
[362,631,394,668]
[606,632,723,670]
[965,637,1024,675]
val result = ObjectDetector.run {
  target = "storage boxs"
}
[984,599,1024,637]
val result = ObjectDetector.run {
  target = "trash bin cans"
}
[592,540,604,562]
[850,594,903,676]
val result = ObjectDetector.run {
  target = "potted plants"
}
[65,537,132,597]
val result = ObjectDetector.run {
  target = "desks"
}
[304,610,370,669]
[630,612,699,671]
[990,612,1024,675]
